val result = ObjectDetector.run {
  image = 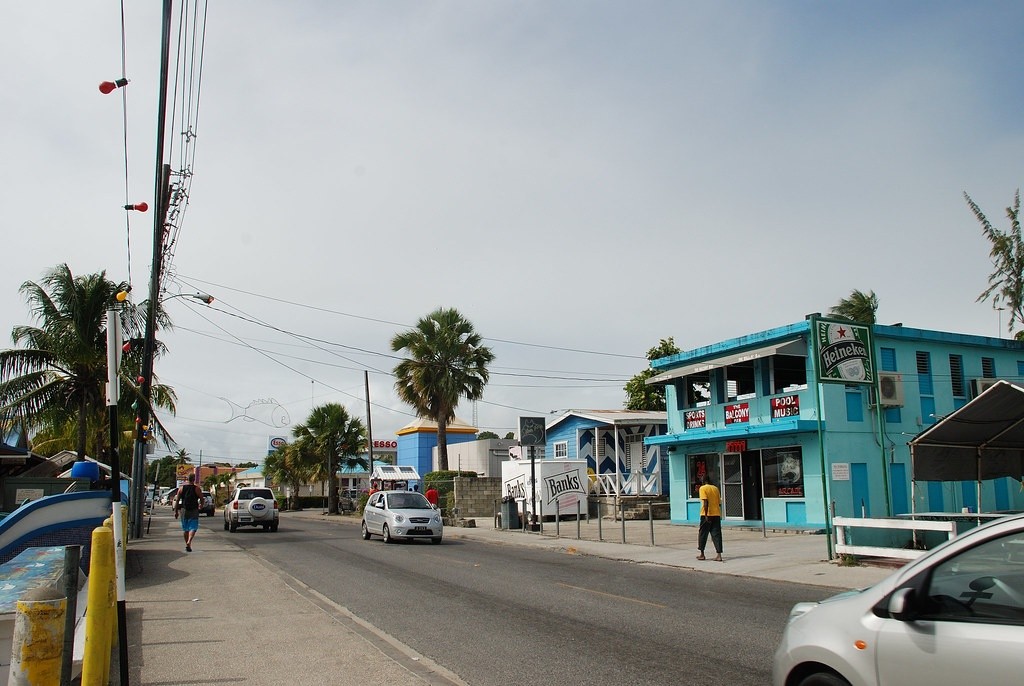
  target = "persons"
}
[696,474,723,562]
[174,474,204,553]
[426,484,438,507]
[369,483,379,496]
[414,485,419,492]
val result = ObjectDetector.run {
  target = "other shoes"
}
[186,543,192,551]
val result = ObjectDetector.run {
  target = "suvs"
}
[223,486,280,533]
[197,491,216,517]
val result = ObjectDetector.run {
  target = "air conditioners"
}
[970,377,1001,399]
[868,373,904,409]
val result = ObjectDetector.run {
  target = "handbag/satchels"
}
[436,507,441,514]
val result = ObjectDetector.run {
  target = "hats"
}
[373,484,377,486]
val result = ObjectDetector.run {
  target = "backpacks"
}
[183,485,198,510]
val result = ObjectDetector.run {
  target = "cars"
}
[144,485,183,511]
[770,510,1024,686]
[361,489,444,545]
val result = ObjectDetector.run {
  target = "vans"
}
[339,488,360,512]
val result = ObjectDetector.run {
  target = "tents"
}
[906,379,1024,552]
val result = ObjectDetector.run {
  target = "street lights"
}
[135,290,213,537]
